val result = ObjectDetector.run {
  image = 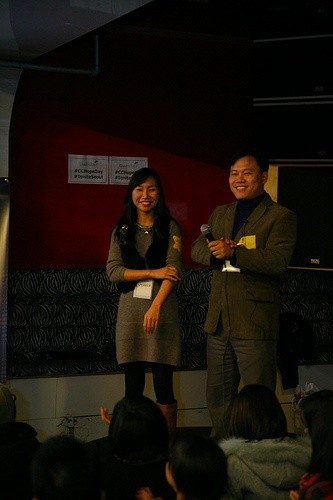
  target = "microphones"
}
[200,224,215,242]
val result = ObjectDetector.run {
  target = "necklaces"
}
[137,224,152,234]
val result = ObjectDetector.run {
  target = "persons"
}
[216,384,313,500]
[87,394,177,500]
[30,435,102,500]
[136,435,227,500]
[0,383,38,500]
[190,149,296,438]
[298,389,333,491]
[107,168,181,455]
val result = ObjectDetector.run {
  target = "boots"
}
[156,400,177,449]
